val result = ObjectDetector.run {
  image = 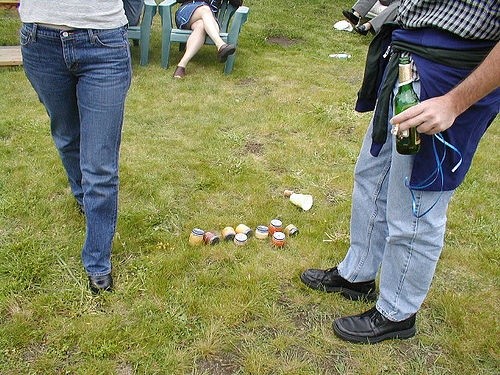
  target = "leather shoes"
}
[300,264,376,302]
[342,10,359,26]
[354,25,370,36]
[216,44,236,64]
[332,302,416,344]
[172,65,186,80]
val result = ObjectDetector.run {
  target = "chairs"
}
[127,0,157,66]
[157,0,250,75]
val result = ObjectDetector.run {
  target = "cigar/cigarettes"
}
[393,123,399,136]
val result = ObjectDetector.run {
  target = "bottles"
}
[394,56,422,155]
[188,219,299,248]
[328,53,352,61]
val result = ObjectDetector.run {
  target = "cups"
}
[289,193,313,211]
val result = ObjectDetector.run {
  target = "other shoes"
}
[87,274,114,296]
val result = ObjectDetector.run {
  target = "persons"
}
[299,0,500,345]
[16,0,237,302]
